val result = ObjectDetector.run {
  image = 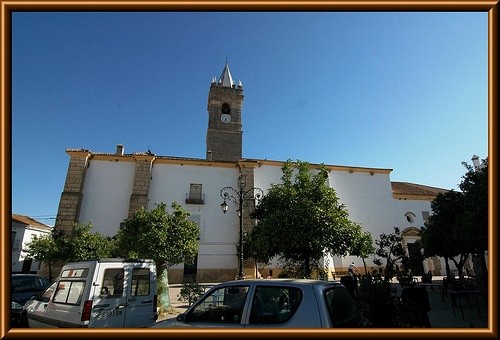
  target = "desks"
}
[417,277,484,324]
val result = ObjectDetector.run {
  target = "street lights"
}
[219,173,265,282]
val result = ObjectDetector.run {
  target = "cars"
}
[9,270,50,323]
[151,278,359,329]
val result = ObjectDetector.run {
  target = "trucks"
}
[27,257,156,329]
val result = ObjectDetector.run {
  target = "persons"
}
[348,262,360,276]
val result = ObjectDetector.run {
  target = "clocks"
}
[220,113,231,124]
